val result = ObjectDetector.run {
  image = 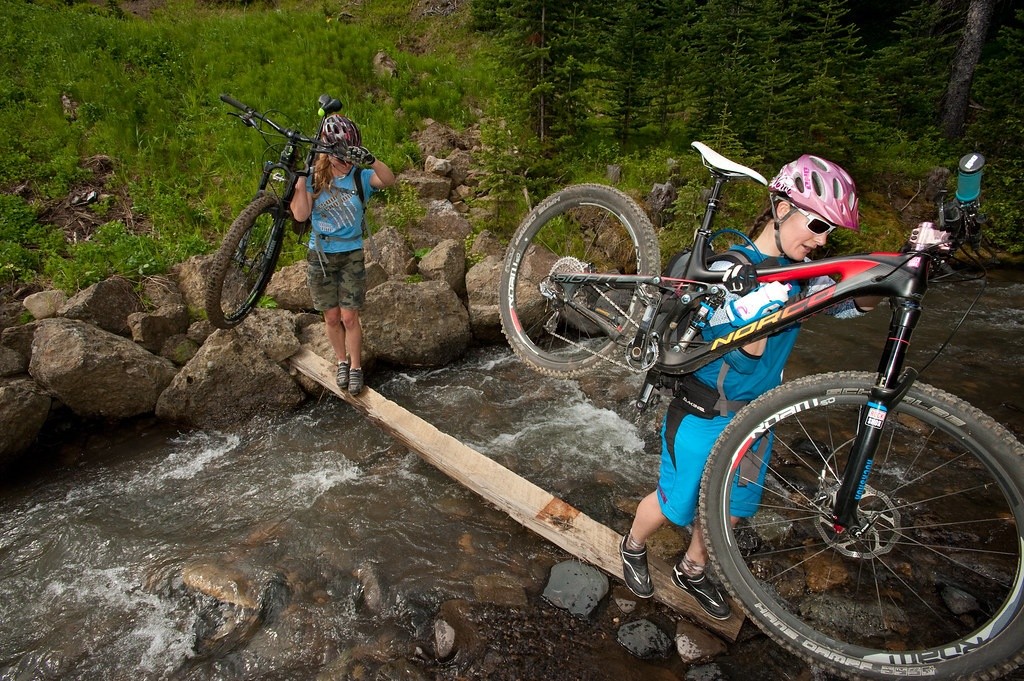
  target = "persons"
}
[617,153,949,620]
[290,113,394,396]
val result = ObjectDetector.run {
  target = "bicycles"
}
[498,141,1024,680]
[205,91,378,331]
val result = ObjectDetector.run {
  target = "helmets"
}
[322,115,360,162]
[768,155,859,232]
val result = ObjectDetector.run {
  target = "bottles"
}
[725,280,792,327]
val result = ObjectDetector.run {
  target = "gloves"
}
[347,145,375,165]
[721,264,759,297]
[296,161,310,177]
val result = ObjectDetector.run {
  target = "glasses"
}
[775,194,838,238]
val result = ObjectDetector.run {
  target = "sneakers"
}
[348,368,364,396]
[336,361,348,388]
[620,533,654,598]
[671,565,732,621]
